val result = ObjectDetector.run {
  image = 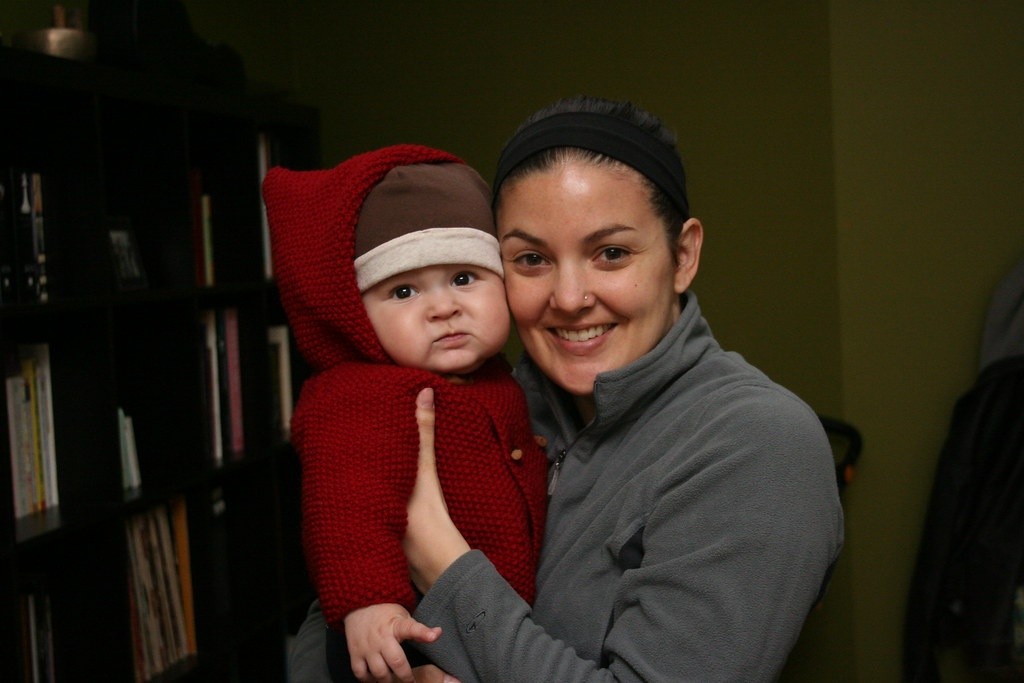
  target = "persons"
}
[256,145,547,683]
[289,95,844,683]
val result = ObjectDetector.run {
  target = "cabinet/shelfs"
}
[0,46,321,683]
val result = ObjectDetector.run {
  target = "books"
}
[0,172,294,683]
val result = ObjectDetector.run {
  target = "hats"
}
[352,161,505,292]
